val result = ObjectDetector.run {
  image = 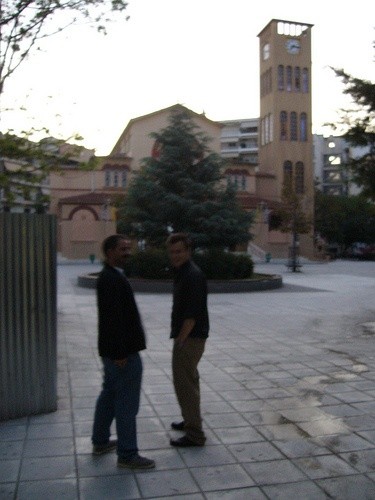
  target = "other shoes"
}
[171,436,203,447]
[171,422,184,429]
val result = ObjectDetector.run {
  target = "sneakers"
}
[117,457,154,468]
[92,439,117,454]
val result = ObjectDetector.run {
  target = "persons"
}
[92,234,157,469]
[167,233,209,448]
